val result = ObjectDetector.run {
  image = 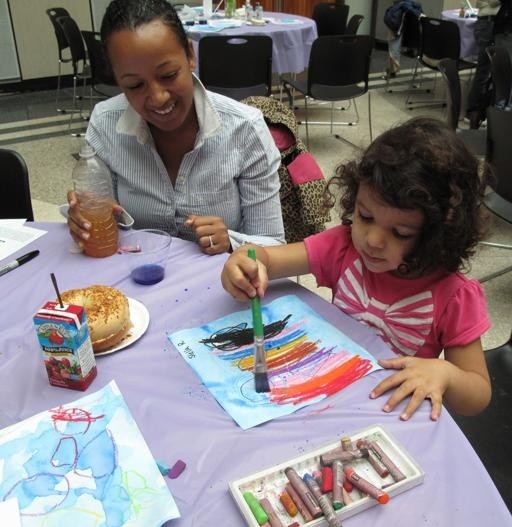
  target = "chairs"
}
[0,149,34,221]
[384,6,512,250]
[443,325,512,515]
[44,1,380,156]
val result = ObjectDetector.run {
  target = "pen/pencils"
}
[243,437,406,527]
[0,250,39,275]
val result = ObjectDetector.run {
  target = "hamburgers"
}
[56,285,130,352]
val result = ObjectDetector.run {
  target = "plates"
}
[92,295,152,356]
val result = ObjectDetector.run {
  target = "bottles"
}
[73,145,125,254]
[459,6,479,18]
[185,0,266,27]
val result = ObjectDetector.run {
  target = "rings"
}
[209,234,214,248]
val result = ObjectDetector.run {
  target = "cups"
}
[120,228,172,284]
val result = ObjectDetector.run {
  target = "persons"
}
[464,0,498,130]
[60,0,286,255]
[219,114,492,420]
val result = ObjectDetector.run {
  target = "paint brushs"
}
[247,247,271,393]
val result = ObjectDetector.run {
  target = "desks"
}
[0,216,512,527]
